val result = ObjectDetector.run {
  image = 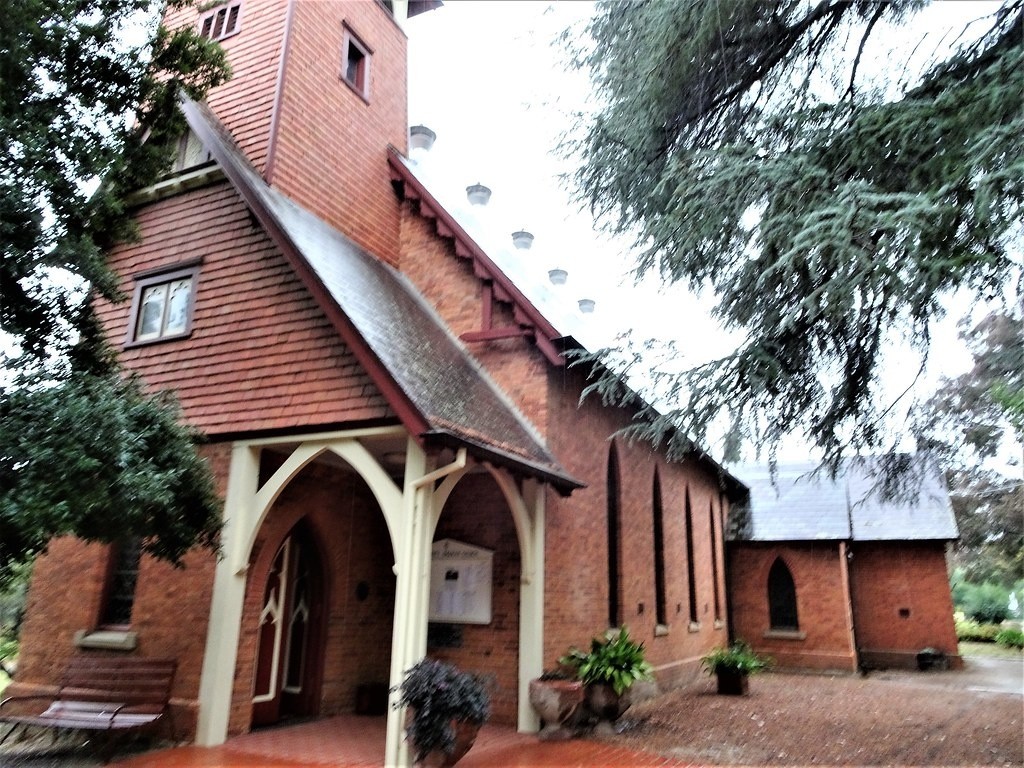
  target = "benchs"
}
[0,658,181,766]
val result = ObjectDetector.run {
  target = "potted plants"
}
[555,623,655,735]
[351,674,388,716]
[388,656,501,768]
[699,638,777,696]
[529,668,585,740]
